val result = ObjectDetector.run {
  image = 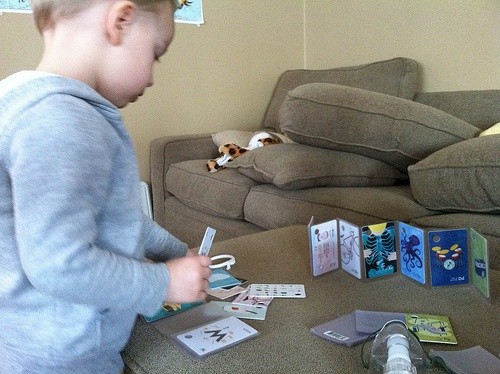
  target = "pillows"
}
[263,57,417,130]
[279,82,482,174]
[407,132,500,212]
[224,142,405,192]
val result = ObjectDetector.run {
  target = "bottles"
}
[369,320,428,374]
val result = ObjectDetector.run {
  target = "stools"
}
[121,223,500,374]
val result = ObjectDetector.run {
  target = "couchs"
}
[149,90,500,250]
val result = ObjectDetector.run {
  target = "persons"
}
[0,0,212,374]
